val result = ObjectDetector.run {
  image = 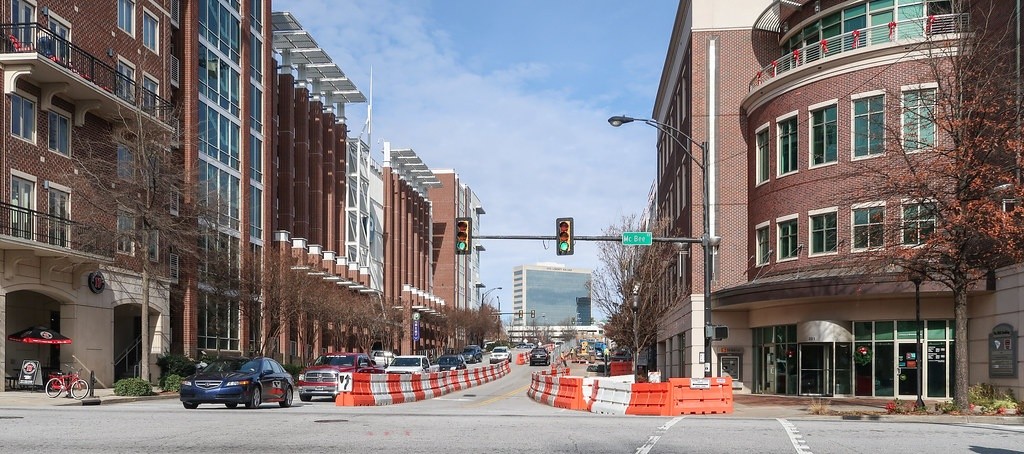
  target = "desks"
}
[13,367,54,390]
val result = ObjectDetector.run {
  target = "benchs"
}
[5,376,46,390]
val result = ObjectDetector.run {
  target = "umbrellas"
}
[7,326,73,359]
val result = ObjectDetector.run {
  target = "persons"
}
[561,356,567,366]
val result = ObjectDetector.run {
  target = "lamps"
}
[41,6,48,15]
[42,181,49,189]
[106,47,114,57]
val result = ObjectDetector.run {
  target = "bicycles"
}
[44,363,90,400]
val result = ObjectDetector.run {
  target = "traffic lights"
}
[456,217,472,255]
[705,325,729,340]
[555,217,574,255]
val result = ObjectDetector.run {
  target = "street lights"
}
[907,276,929,408]
[479,285,504,352]
[607,114,714,377]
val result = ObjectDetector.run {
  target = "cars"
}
[435,353,466,371]
[384,355,432,375]
[370,350,398,368]
[179,356,295,409]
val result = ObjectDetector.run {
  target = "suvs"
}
[462,344,483,364]
[489,345,512,364]
[298,351,378,402]
[529,347,551,365]
